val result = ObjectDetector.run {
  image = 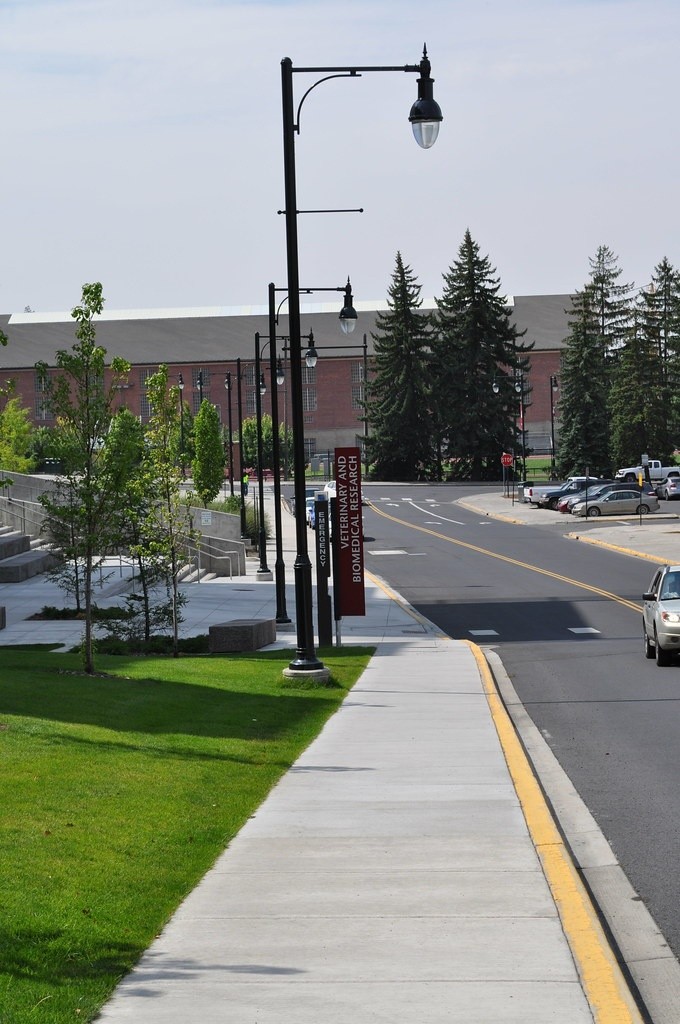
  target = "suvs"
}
[642,564,680,667]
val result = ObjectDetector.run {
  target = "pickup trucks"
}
[524,477,598,509]
[615,460,680,483]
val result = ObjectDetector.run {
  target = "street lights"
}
[196,368,203,404]
[268,275,358,631]
[255,327,318,581]
[237,357,266,546]
[224,371,234,496]
[549,375,558,482]
[277,42,443,684]
[179,372,185,477]
[492,373,526,481]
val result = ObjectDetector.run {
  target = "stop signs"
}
[501,454,513,466]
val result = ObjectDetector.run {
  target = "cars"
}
[290,487,319,516]
[559,484,602,512]
[305,497,314,523]
[539,480,613,511]
[572,490,660,517]
[567,481,655,509]
[324,480,364,503]
[655,477,680,501]
[309,505,315,529]
